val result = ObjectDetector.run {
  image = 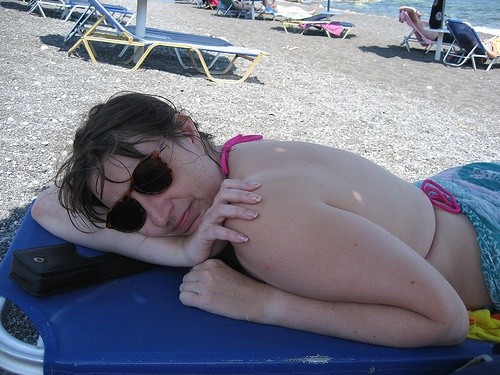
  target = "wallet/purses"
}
[7,243,144,299]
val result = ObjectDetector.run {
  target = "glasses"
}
[105,146,173,233]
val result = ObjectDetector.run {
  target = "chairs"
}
[398,6,458,54]
[0,198,500,375]
[22,0,355,83]
[443,19,500,71]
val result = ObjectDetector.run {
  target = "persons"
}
[207,0,219,8]
[31,91,500,346]
[461,22,500,60]
[402,6,456,46]
[231,0,278,15]
